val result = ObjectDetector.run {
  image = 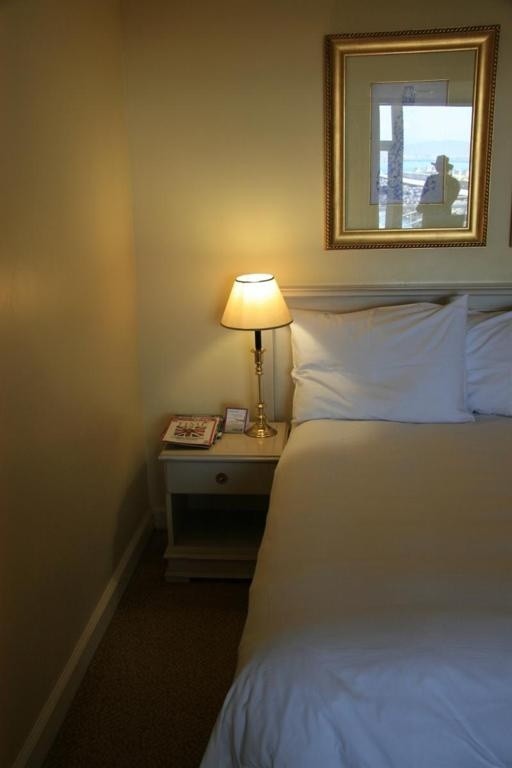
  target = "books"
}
[160,415,224,450]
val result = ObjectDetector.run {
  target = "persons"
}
[415,153,460,228]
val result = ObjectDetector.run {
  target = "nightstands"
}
[156,419,290,585]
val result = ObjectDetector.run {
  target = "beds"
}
[212,281,512,768]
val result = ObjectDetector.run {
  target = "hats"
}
[431,155,454,169]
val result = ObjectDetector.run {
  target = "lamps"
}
[219,272,294,439]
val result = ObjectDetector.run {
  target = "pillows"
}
[284,294,511,425]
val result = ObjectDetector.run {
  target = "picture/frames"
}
[322,22,504,252]
